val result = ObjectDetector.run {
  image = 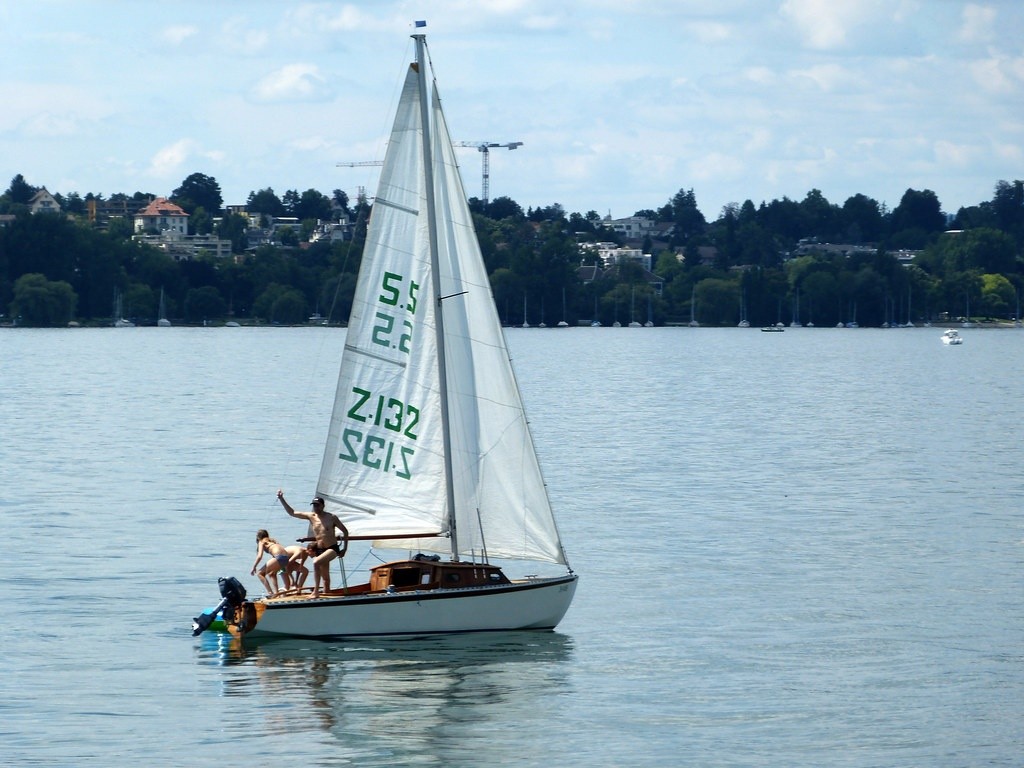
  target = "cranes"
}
[448,139,524,213]
[333,160,383,166]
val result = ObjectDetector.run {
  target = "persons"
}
[277,489,348,599]
[251,530,289,598]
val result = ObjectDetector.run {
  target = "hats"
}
[309,497,325,505]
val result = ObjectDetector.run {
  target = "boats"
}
[941,328,963,346]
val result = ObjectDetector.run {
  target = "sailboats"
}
[250,11,579,643]
